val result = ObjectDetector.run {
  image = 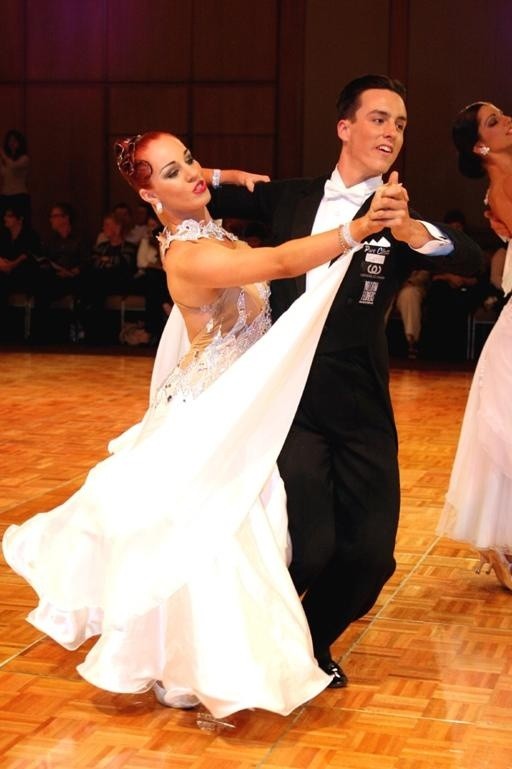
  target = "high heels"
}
[474,548,512,594]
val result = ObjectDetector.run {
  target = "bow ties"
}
[322,177,369,209]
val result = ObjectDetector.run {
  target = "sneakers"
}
[150,681,200,709]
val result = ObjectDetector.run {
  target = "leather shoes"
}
[321,657,348,690]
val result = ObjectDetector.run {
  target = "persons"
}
[383,208,512,362]
[114,127,414,719]
[1,127,298,355]
[136,74,487,691]
[438,99,512,598]
